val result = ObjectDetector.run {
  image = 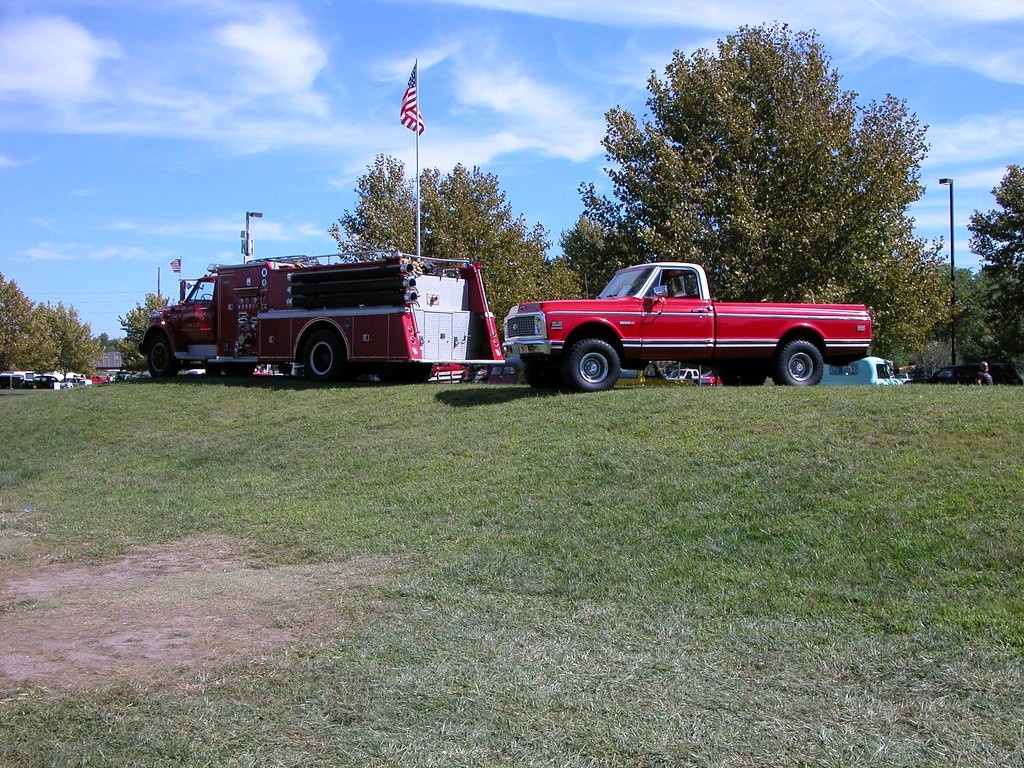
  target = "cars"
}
[814,355,895,386]
[894,372,913,384]
[486,357,524,385]
[697,370,723,385]
[0,368,152,391]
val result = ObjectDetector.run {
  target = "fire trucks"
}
[137,249,506,381]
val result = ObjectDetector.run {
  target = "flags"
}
[400,58,425,136]
[170,259,182,273]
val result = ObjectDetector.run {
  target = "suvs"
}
[926,362,1023,386]
[664,369,701,384]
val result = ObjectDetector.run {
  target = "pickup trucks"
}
[611,359,696,387]
[501,260,877,394]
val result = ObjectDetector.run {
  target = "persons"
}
[887,357,933,380]
[975,362,993,386]
[254,365,274,376]
[81,372,113,383]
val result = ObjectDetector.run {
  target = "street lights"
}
[245,210,263,261]
[939,177,958,366]
